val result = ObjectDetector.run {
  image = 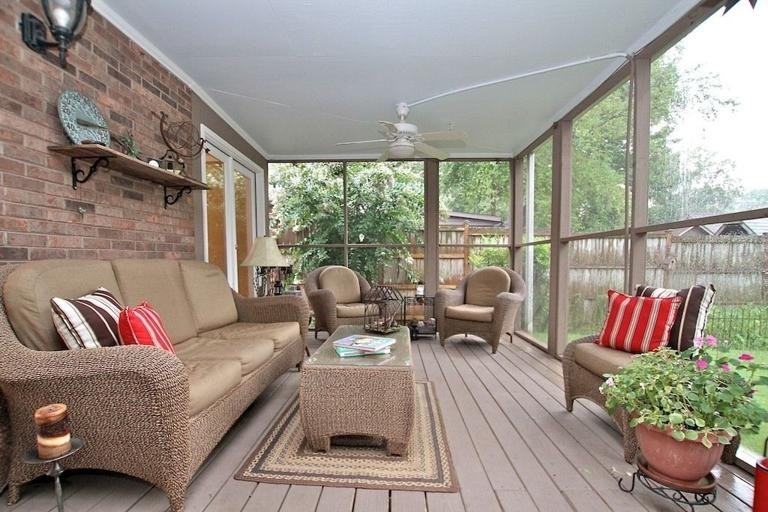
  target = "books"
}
[332,346,391,359]
[334,331,397,351]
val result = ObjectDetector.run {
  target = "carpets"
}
[233,380,462,494]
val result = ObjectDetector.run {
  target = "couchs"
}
[0,258,311,509]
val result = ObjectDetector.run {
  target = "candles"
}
[34,403,72,458]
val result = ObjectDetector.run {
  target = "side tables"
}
[403,296,437,340]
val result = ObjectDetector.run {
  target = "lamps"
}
[21,1,92,67]
[240,236,290,296]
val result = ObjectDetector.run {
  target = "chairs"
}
[561,281,714,462]
[306,266,398,336]
[433,261,528,355]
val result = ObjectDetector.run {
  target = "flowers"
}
[592,335,766,445]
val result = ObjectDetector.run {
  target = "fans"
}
[333,100,463,165]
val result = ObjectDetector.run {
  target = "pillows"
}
[597,283,714,358]
[48,280,175,353]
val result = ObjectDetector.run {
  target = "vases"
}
[631,411,730,484]
